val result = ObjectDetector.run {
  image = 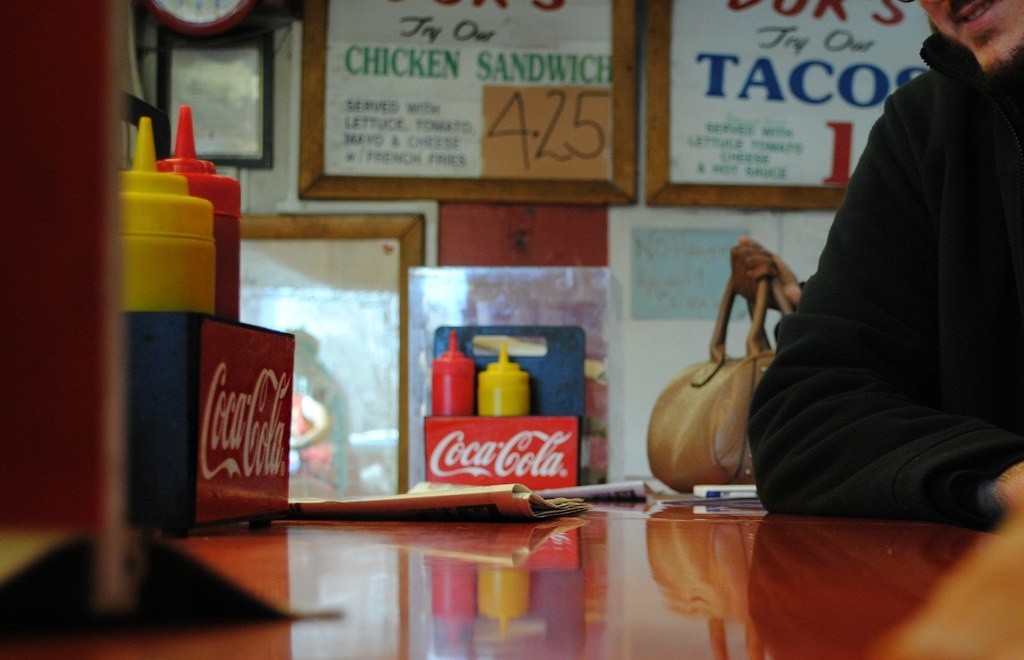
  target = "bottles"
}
[477,566,531,641]
[431,331,474,418]
[117,117,214,532]
[157,107,240,321]
[431,560,474,649]
[477,343,530,417]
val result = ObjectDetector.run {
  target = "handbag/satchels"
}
[647,273,794,492]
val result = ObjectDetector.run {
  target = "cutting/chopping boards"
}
[434,327,586,422]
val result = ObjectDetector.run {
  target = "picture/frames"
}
[237,213,427,498]
[157,27,274,169]
[301,0,640,205]
[645,0,937,208]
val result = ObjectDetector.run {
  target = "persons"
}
[730,0,1024,531]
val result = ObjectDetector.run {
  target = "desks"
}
[1,499,996,660]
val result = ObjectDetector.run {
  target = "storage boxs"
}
[121,310,296,534]
